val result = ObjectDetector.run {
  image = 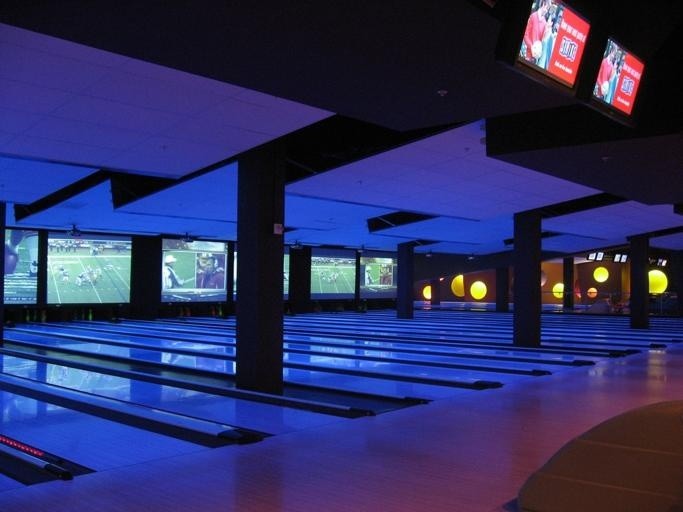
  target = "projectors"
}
[184,237,193,242]
[71,231,81,236]
[294,244,303,249]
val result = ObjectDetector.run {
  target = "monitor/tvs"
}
[576,35,647,129]
[494,0,593,98]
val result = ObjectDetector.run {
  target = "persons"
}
[519,0,564,70]
[593,43,626,104]
